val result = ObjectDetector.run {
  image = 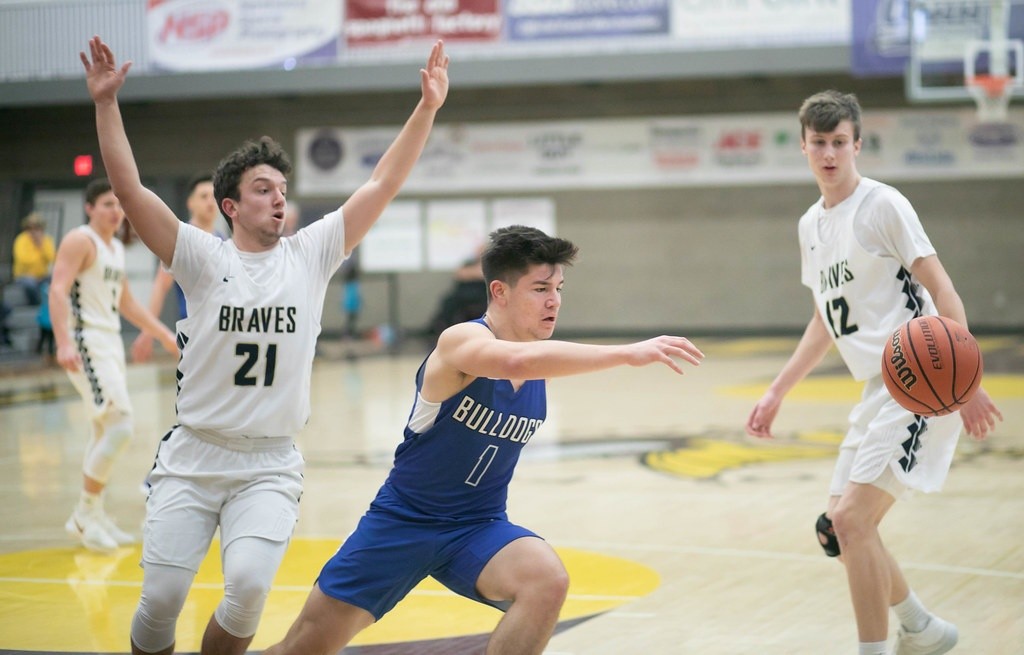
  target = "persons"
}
[79,32,450,655]
[30,273,60,367]
[130,171,218,367]
[263,224,707,655]
[746,91,1006,655]
[13,210,56,307]
[48,179,179,553]
[419,241,488,353]
[342,265,362,336]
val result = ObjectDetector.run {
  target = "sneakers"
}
[891,613,957,655]
[63,508,134,553]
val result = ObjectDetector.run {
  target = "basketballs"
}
[881,315,983,417]
